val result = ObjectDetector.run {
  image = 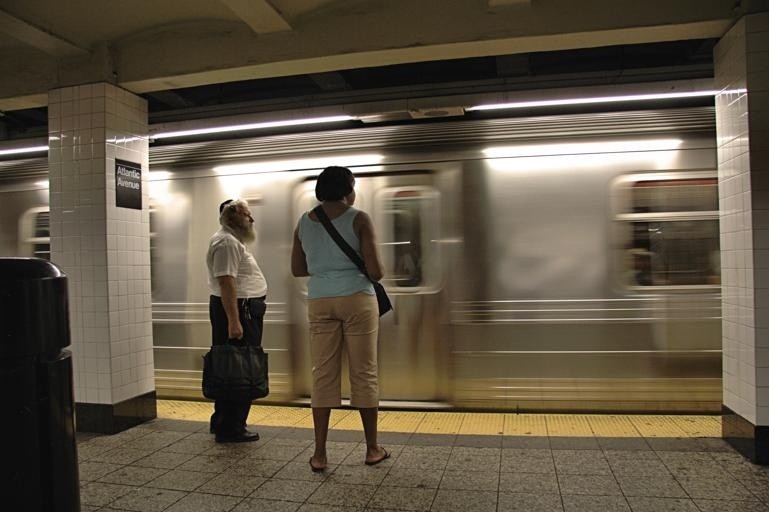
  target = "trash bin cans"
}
[0,255,83,512]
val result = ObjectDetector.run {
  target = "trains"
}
[2,103,723,417]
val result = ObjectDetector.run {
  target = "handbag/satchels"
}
[199,337,271,402]
[371,278,394,318]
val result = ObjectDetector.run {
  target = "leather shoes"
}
[215,428,260,443]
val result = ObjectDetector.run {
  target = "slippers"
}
[363,443,392,466]
[309,453,328,472]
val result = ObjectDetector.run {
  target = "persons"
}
[394,213,420,286]
[695,235,721,284]
[626,204,668,284]
[292,167,390,471]
[206,199,268,443]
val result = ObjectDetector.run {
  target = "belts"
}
[209,293,267,304]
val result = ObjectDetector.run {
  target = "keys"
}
[243,306,251,319]
